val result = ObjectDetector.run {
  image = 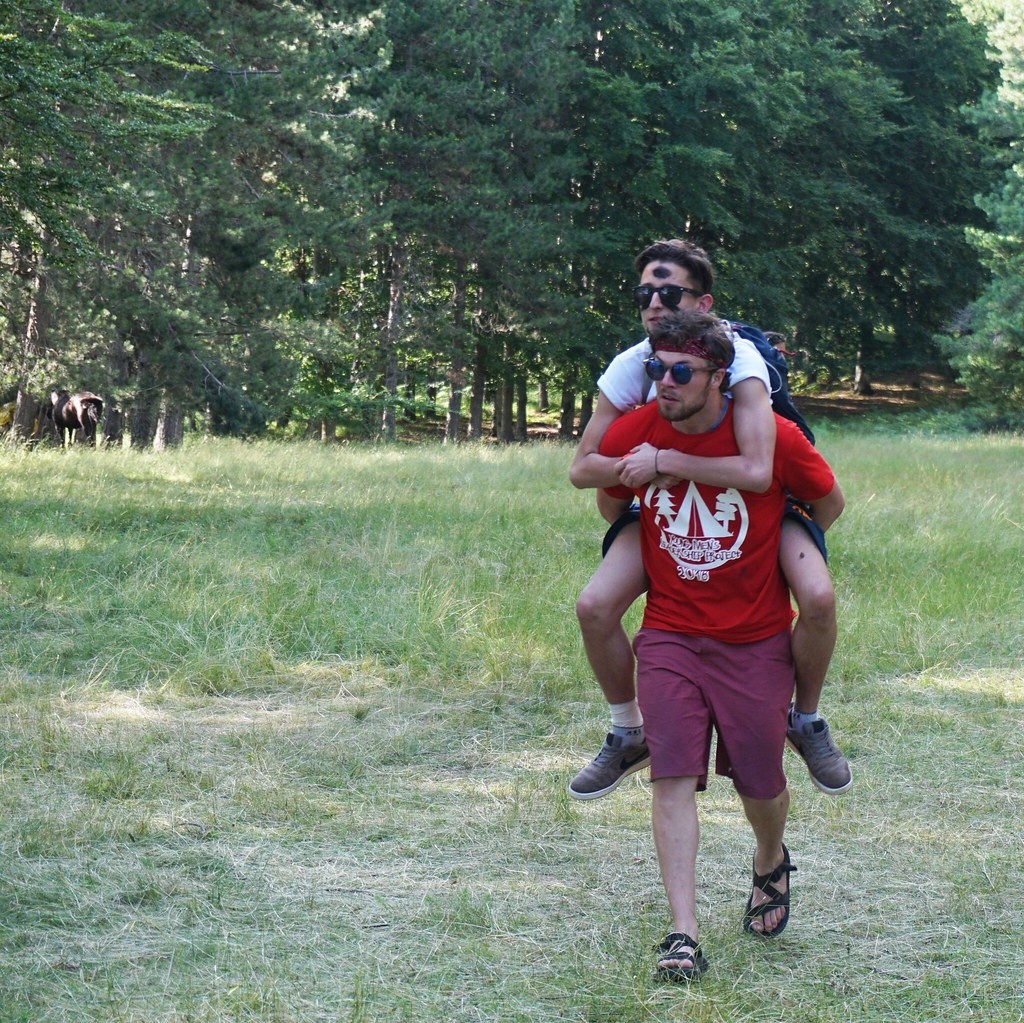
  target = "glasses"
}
[632,283,704,309]
[642,356,724,385]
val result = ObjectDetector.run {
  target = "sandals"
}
[652,932,710,982]
[743,843,797,938]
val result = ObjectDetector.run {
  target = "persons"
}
[568,240,856,801]
[594,308,845,979]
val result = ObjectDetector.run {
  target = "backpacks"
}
[731,320,815,446]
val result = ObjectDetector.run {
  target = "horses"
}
[47,389,103,448]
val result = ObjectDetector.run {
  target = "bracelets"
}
[654,449,661,476]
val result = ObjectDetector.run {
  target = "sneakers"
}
[786,707,854,795]
[567,733,653,802]
